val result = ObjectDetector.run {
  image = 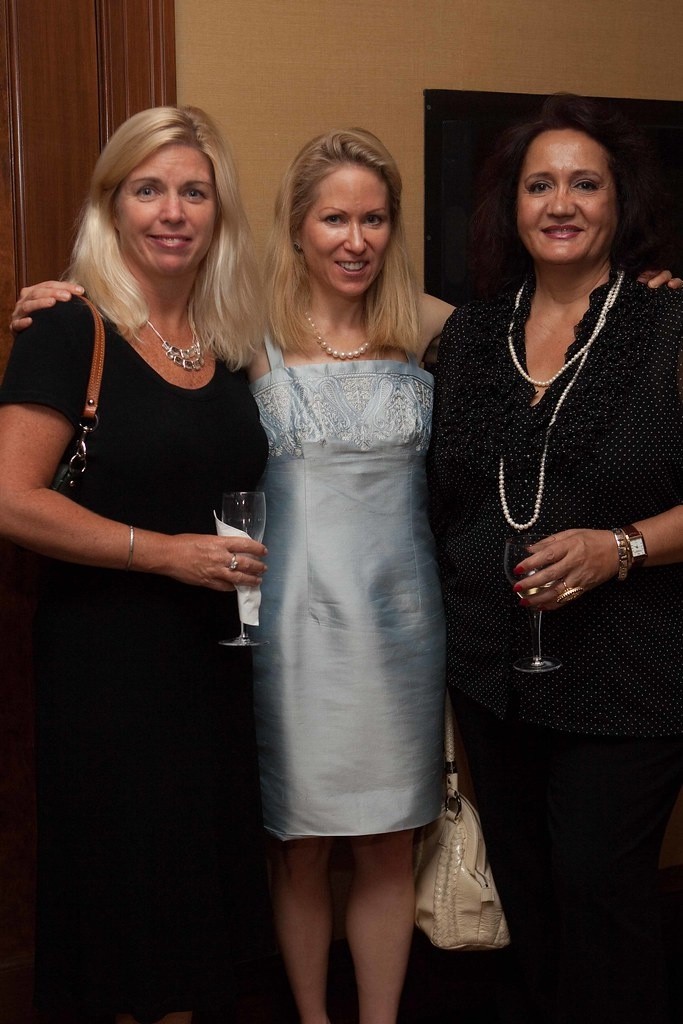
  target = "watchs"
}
[622,524,648,571]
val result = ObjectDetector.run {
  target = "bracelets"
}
[613,528,629,580]
[125,524,134,569]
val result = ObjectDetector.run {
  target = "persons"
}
[424,89,682,1023]
[0,105,272,1024]
[10,128,682,1024]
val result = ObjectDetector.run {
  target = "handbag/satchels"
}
[1,293,106,598]
[414,687,510,953]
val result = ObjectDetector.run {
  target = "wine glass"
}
[505,535,562,672]
[219,491,268,648]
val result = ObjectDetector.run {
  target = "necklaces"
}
[147,321,206,372]
[497,270,624,530]
[304,311,370,360]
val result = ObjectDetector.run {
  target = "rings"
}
[556,577,584,604]
[229,553,238,571]
